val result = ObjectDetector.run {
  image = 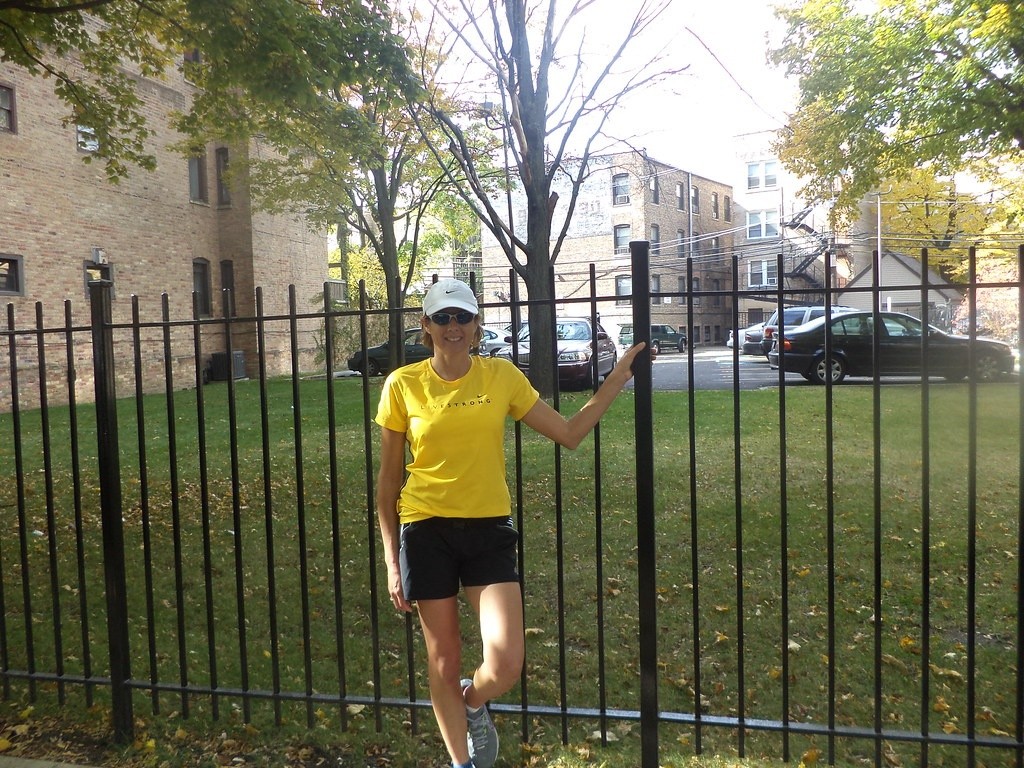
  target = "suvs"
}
[760,304,929,364]
[619,323,687,355]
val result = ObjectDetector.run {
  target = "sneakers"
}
[459,678,499,768]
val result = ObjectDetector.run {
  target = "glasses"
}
[428,311,475,326]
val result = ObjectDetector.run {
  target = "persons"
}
[374,280,657,768]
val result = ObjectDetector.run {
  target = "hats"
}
[422,278,479,317]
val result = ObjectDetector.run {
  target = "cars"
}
[497,318,616,388]
[727,321,767,356]
[482,325,512,359]
[768,310,1015,386]
[505,321,527,334]
[348,327,435,377]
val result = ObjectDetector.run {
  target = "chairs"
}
[572,327,588,340]
[866,317,874,336]
